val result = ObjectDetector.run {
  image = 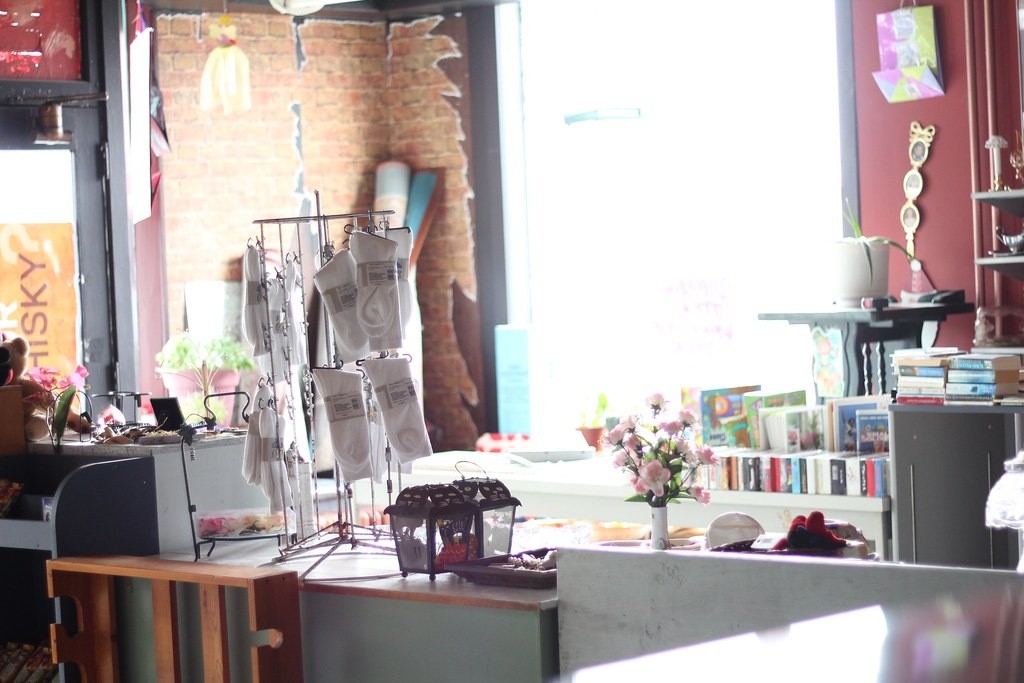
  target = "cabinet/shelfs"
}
[969,189,1024,284]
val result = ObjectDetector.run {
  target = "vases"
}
[649,506,671,551]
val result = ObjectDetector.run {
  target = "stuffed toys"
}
[0,336,56,441]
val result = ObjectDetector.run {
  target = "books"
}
[698,383,890,501]
[888,346,1023,408]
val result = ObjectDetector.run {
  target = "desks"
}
[104,523,560,683]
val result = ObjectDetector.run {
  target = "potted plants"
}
[838,197,915,309]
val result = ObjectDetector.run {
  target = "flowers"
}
[603,392,721,508]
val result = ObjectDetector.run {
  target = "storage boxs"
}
[889,404,1024,572]
